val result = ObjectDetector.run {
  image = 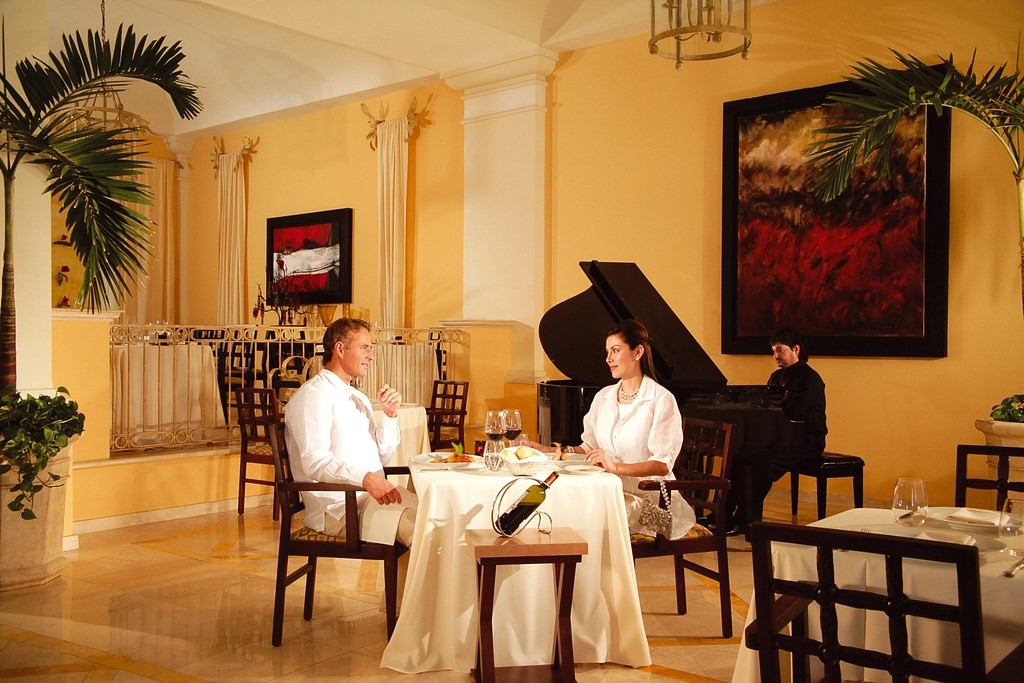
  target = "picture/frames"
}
[720,64,951,358]
[266,208,353,306]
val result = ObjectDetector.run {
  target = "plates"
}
[917,507,1022,532]
[412,451,484,469]
[832,526,1007,557]
[565,465,604,475]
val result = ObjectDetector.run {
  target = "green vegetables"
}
[452,442,463,458]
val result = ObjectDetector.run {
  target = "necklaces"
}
[620,384,640,400]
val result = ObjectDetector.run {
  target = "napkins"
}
[915,530,976,547]
[360,485,418,545]
[947,508,1011,527]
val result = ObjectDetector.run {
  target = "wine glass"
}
[110,320,185,347]
[484,409,522,453]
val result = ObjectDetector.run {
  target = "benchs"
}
[792,451,865,520]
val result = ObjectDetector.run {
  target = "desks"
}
[316,341,442,426]
[110,337,229,453]
[466,526,588,683]
[372,401,432,490]
[379,451,652,674]
[730,508,1024,683]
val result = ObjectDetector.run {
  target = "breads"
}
[502,446,544,461]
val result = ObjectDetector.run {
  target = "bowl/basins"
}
[504,462,546,476]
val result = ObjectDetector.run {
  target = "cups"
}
[892,476,928,526]
[483,433,529,472]
[999,499,1024,557]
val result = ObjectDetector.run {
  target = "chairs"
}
[158,328,310,435]
[232,377,1024,683]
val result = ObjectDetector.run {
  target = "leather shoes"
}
[699,512,734,525]
[726,519,745,536]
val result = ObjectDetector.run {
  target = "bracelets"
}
[562,446,575,453]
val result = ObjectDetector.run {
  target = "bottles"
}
[495,470,559,535]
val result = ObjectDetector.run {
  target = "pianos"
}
[536,260,806,528]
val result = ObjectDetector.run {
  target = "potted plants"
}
[974,394,1024,515]
[0,21,206,592]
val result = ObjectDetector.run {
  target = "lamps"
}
[648,0,752,71]
[31,0,186,169]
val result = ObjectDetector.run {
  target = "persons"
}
[284,318,417,618]
[515,320,697,541]
[694,327,828,537]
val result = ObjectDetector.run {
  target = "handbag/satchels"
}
[638,478,673,539]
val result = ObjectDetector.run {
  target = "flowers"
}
[53,234,71,308]
